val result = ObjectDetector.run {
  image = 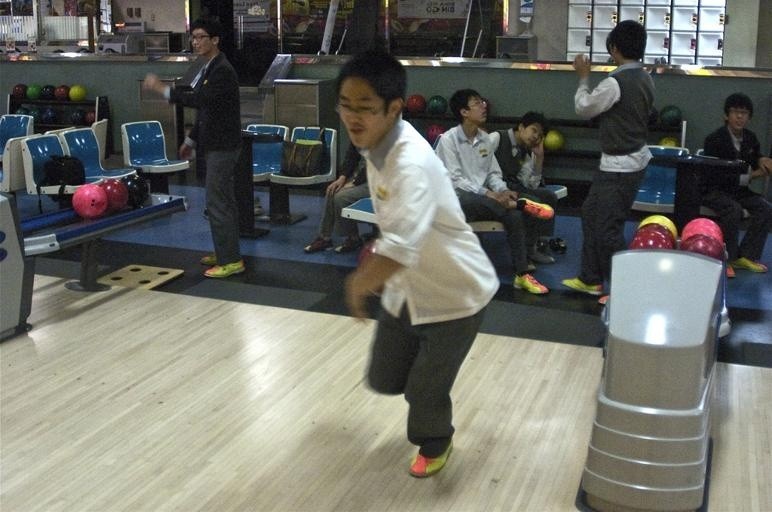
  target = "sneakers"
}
[408,439,454,478]
[204,259,245,278]
[561,277,602,297]
[304,237,333,252]
[726,263,736,278]
[334,238,363,253]
[513,249,555,305]
[731,256,769,273]
[518,197,555,221]
[200,253,218,265]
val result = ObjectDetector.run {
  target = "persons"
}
[487,111,556,264]
[304,141,374,254]
[559,19,657,306]
[332,48,501,479]
[141,16,246,280]
[696,91,772,279]
[433,88,556,296]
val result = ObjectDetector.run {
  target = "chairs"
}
[242,122,336,225]
[629,142,753,221]
[340,131,568,275]
[0,115,189,203]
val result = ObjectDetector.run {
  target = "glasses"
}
[335,103,383,118]
[469,100,486,108]
[188,34,209,42]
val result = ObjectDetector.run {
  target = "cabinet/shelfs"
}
[144,31,171,55]
[274,78,340,137]
[7,94,108,133]
[496,36,537,63]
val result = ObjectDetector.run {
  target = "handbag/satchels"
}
[36,154,84,186]
[281,138,323,177]
